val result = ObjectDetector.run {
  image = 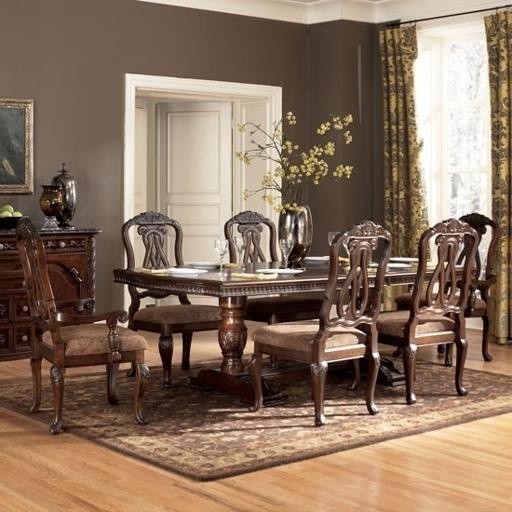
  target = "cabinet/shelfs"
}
[0,227,102,361]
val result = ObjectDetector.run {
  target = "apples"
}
[0,204,23,217]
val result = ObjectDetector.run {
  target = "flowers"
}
[235,109,355,214]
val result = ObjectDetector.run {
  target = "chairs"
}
[349,218,479,405]
[392,212,501,367]
[247,219,393,426]
[16,216,151,435]
[225,210,280,325]
[121,210,222,390]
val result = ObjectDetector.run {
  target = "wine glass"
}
[280,238,296,269]
[215,239,230,275]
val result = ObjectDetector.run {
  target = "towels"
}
[151,267,208,274]
[230,272,278,280]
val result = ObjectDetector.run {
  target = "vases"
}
[278,205,314,270]
[39,185,64,231]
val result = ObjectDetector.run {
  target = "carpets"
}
[0,355,512,482]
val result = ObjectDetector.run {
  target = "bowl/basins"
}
[0,216,22,230]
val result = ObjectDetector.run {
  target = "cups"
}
[328,231,341,247]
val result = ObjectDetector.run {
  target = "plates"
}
[165,262,219,278]
[256,269,303,275]
[371,258,419,269]
[302,256,331,262]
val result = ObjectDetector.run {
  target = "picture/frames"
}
[0,97,34,194]
